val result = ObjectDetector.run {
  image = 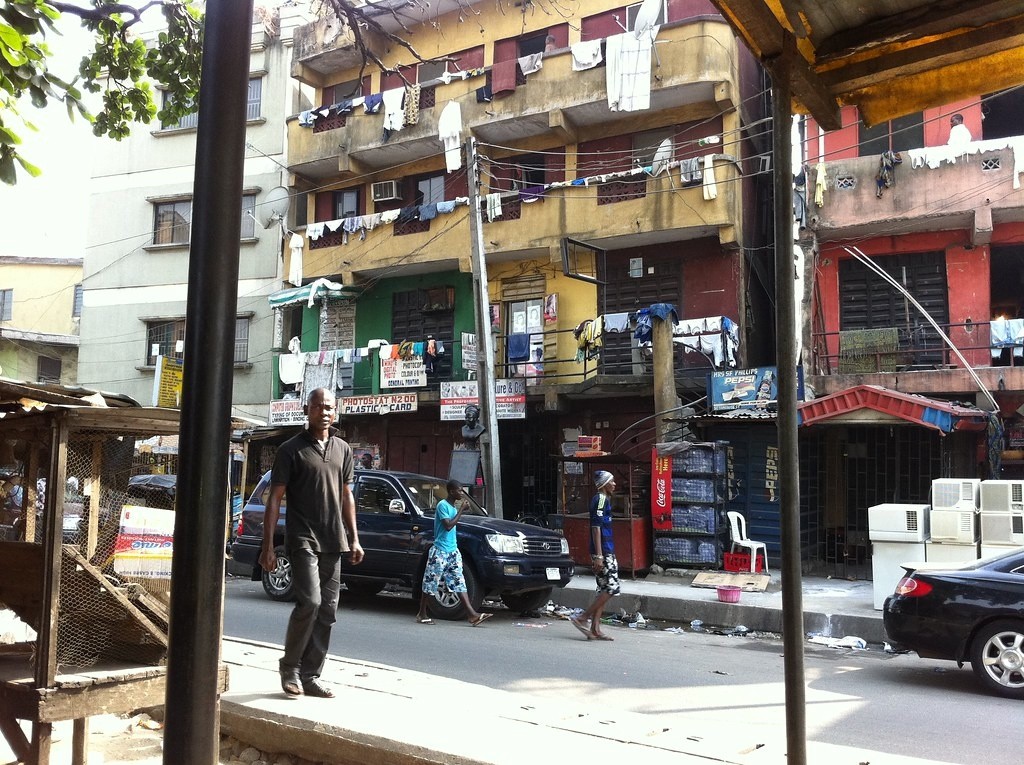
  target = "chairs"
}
[726,511,769,573]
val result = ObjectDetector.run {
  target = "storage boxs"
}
[725,552,763,572]
[577,436,602,452]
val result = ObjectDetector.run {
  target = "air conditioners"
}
[370,181,403,201]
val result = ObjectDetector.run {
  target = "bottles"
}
[755,370,773,409]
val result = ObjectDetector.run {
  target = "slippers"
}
[416,618,435,625]
[473,613,493,626]
[572,621,596,640]
[587,633,614,641]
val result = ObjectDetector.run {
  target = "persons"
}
[572,471,621,640]
[262,388,363,700]
[361,454,377,470]
[416,480,493,626]
[0,474,22,509]
[946,114,971,145]
[462,405,487,450]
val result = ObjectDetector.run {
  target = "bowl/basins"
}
[716,586,742,602]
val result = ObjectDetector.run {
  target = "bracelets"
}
[596,555,603,559]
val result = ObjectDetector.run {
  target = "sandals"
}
[304,678,335,698]
[281,673,304,699]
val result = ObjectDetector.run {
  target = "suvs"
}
[223,471,574,620]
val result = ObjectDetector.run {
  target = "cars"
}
[881,545,1023,700]
[62,502,85,536]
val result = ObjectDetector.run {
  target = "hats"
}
[592,470,614,490]
